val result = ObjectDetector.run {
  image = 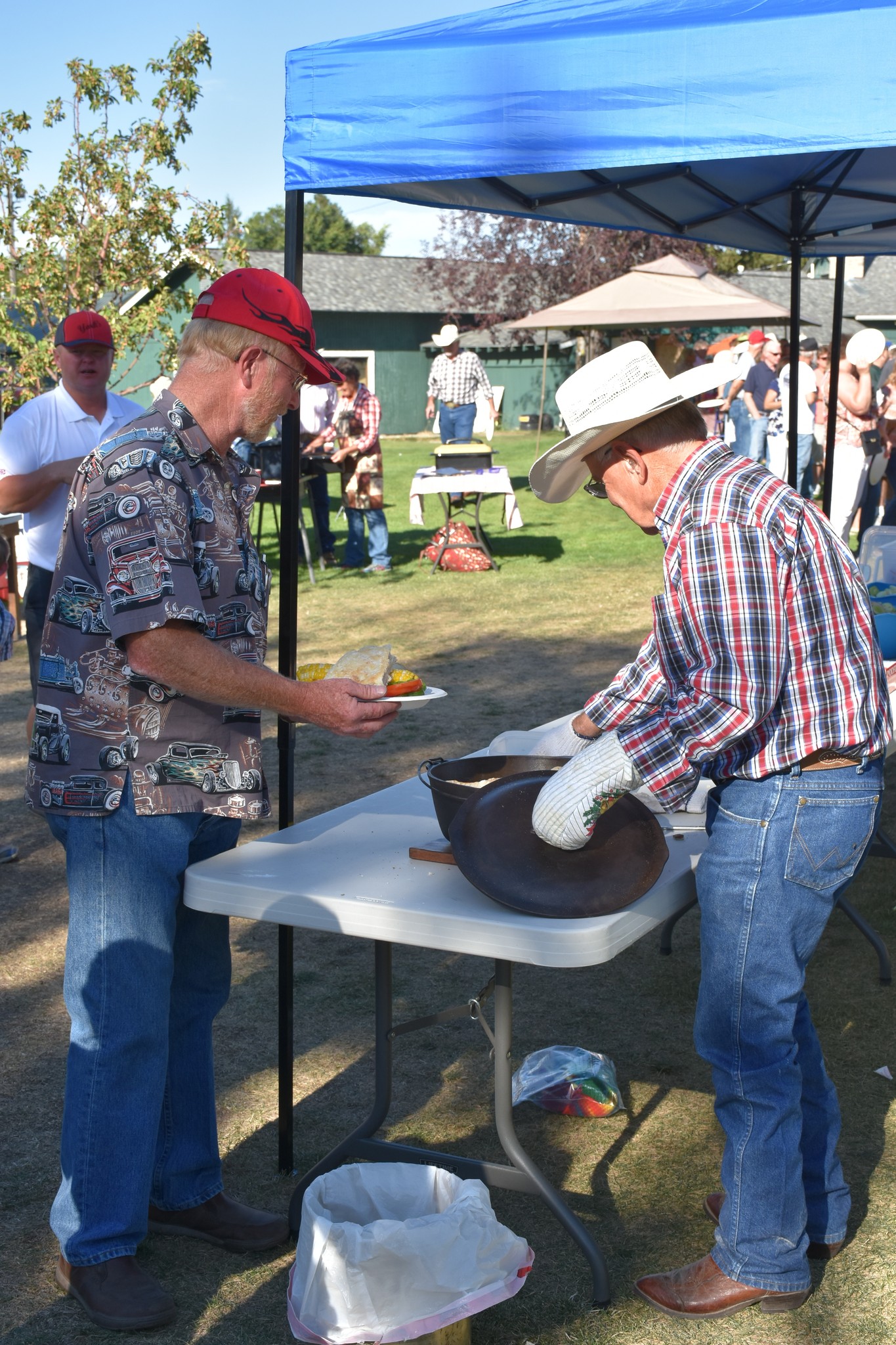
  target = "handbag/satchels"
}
[860,429,882,456]
[422,521,494,572]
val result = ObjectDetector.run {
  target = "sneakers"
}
[362,564,393,573]
[322,552,337,565]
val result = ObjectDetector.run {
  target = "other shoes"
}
[450,496,463,508]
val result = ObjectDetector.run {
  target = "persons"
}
[528,340,894,1321]
[274,383,339,567]
[720,331,768,461]
[777,338,820,506]
[0,311,147,713]
[424,324,500,508]
[302,358,394,574]
[23,268,402,1333]
[821,333,895,548]
[715,330,748,456]
[743,339,781,473]
[763,340,790,486]
[814,344,830,498]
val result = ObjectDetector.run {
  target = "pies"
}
[323,644,398,686]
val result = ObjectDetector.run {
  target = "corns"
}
[296,663,420,682]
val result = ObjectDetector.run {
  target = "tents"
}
[276,0,864,1176]
[501,253,822,336]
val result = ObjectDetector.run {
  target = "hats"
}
[748,330,769,344]
[799,338,823,352]
[432,325,466,347]
[54,311,116,352]
[192,268,346,385]
[528,340,746,505]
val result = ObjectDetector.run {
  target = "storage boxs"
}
[431,436,499,471]
[256,435,284,480]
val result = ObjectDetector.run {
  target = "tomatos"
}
[386,679,423,696]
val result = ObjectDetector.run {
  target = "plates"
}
[697,399,725,408]
[354,686,448,711]
[845,328,886,366]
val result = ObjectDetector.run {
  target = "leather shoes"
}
[146,1190,290,1254]
[633,1252,812,1320]
[703,1194,843,1259]
[56,1252,176,1331]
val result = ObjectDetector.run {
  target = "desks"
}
[408,465,525,574]
[181,707,896,1307]
[255,472,325,585]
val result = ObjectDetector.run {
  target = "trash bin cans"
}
[285,1161,536,1344]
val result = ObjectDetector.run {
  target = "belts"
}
[444,402,460,409]
[791,744,883,772]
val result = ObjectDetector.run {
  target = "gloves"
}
[532,728,647,851]
[532,714,603,758]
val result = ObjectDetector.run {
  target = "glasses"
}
[583,446,643,498]
[819,357,828,360]
[769,352,782,357]
[235,350,308,393]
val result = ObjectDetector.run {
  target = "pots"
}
[416,756,669,919]
